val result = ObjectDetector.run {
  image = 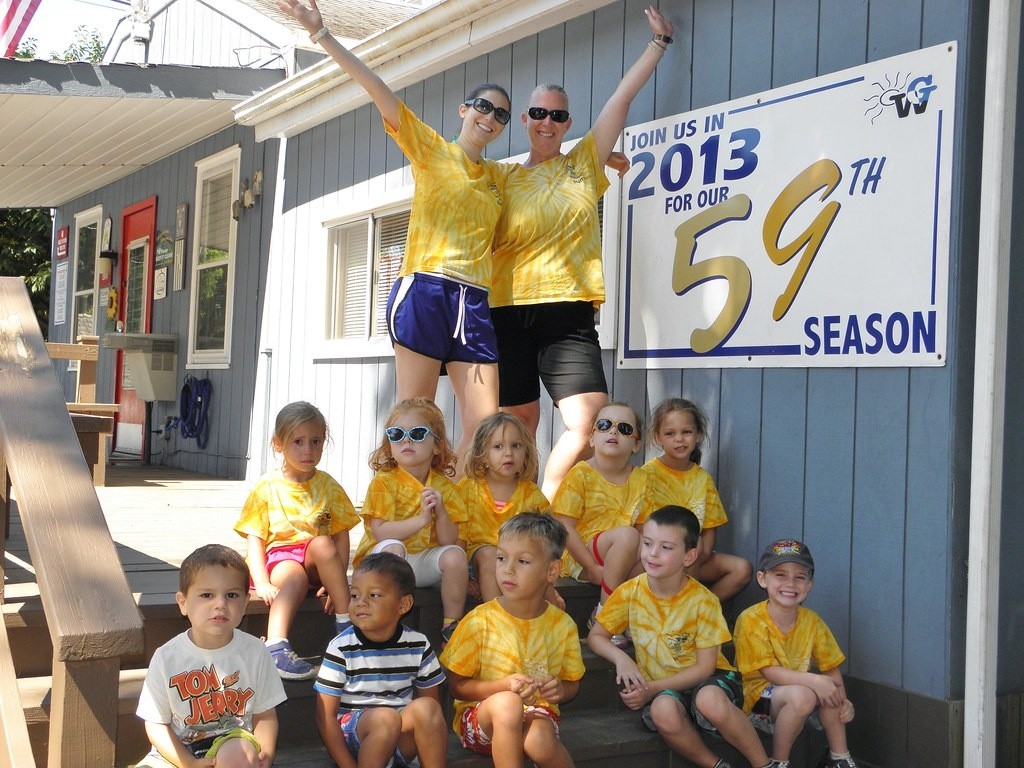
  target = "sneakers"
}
[587,608,629,648]
[771,758,789,768]
[259,636,316,679]
[821,748,858,768]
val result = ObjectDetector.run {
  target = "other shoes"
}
[441,619,461,642]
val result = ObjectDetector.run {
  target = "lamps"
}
[99,250,118,274]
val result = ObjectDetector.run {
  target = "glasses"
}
[385,426,441,443]
[591,418,639,440]
[464,98,511,126]
[525,107,570,122]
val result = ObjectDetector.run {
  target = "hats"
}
[757,538,814,579]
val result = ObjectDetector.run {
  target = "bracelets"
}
[309,26,329,44]
[652,33,673,45]
[647,40,667,56]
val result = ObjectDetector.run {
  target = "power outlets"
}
[159,425,170,439]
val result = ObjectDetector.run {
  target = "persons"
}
[277,0,630,485]
[483,6,673,505]
[134,398,858,768]
